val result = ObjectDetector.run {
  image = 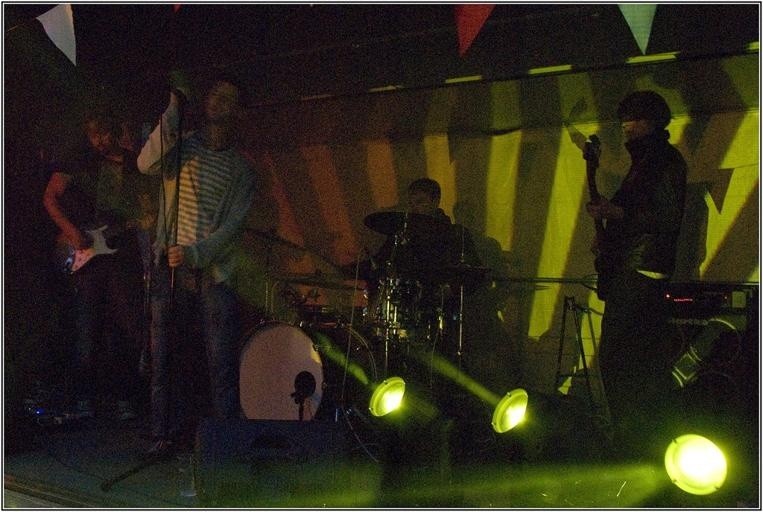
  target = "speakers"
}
[664,282,759,428]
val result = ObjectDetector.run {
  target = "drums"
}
[237,318,377,421]
[285,303,335,328]
[366,257,460,350]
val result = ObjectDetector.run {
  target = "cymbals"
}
[237,226,306,252]
[363,211,450,241]
[269,272,365,292]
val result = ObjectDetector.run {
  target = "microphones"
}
[171,88,187,105]
[366,248,379,272]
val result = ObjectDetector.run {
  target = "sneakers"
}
[54,398,140,422]
[137,434,170,458]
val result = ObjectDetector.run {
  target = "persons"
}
[342,179,486,296]
[131,72,257,460]
[41,107,158,445]
[585,90,686,465]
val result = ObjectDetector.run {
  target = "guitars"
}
[47,211,159,282]
[583,135,617,300]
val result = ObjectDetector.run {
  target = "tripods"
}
[102,100,192,492]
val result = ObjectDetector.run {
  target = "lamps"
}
[661,412,730,497]
[369,374,461,444]
[488,385,585,460]
